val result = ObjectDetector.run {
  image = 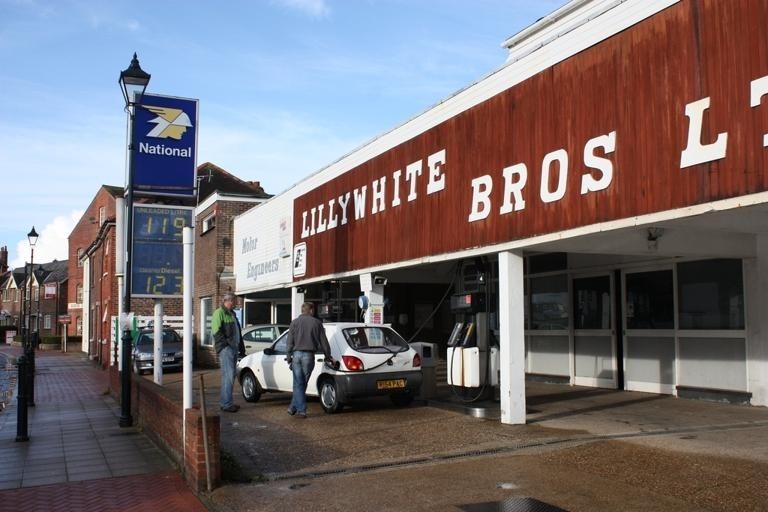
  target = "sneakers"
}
[287,409,306,421]
[221,406,240,412]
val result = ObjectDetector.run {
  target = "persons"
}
[210,292,246,412]
[286,302,339,419]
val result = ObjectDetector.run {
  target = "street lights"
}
[25,224,39,345]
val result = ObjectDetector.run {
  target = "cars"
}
[132,327,182,375]
[235,321,424,413]
[238,323,291,360]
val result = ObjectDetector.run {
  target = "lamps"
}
[638,227,666,252]
